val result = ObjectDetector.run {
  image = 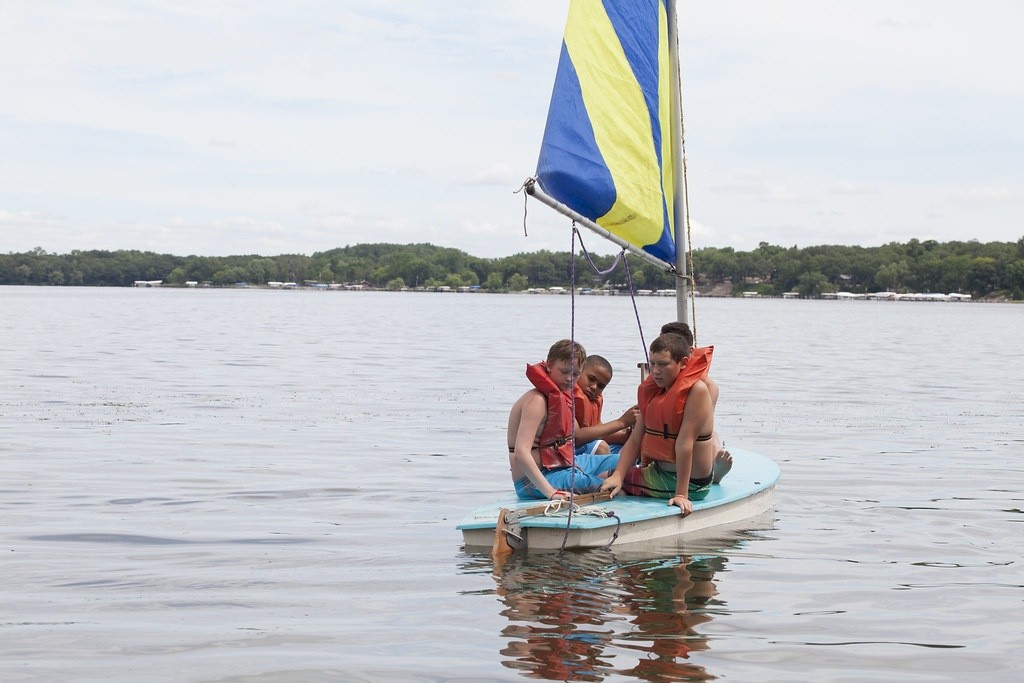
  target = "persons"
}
[601,332,714,518]
[508,339,620,500]
[660,322,732,484]
[574,355,640,455]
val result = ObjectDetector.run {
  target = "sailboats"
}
[456,0,780,559]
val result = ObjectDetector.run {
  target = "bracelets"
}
[551,489,565,500]
